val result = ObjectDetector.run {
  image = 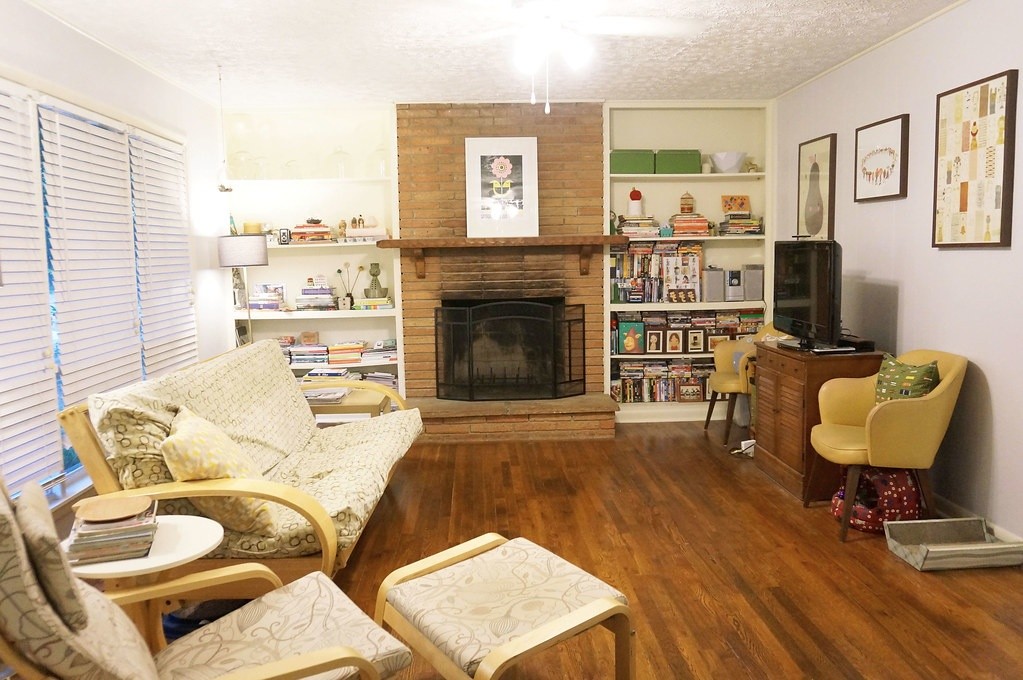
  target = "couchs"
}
[57,337,422,594]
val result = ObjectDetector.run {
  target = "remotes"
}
[812,347,856,355]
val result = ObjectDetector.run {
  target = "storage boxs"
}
[744,265,764,300]
[656,149,701,174]
[702,267,724,303]
[609,150,655,173]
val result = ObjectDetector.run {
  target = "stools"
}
[373,531,638,680]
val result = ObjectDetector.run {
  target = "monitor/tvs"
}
[773,240,842,351]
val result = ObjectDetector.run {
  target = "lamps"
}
[216,234,269,344]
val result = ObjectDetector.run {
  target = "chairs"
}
[803,347,968,541]
[0,467,412,680]
[704,322,797,446]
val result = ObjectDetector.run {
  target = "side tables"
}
[52,515,224,656]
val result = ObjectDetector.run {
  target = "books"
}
[275,336,398,406]
[294,295,394,310]
[65,498,158,565]
[609,195,763,402]
[287,224,389,245]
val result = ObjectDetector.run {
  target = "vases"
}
[346,294,354,305]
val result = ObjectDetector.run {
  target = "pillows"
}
[875,352,938,411]
[154,406,275,539]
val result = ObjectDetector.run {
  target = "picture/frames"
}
[854,113,910,202]
[677,384,702,403]
[685,327,705,353]
[617,321,645,353]
[703,377,730,401]
[796,132,837,239]
[665,328,685,353]
[735,332,758,342]
[705,334,731,352]
[465,136,538,239]
[644,327,664,354]
[931,69,1018,247]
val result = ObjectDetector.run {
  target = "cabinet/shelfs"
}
[239,241,400,409]
[751,339,892,507]
[611,172,770,403]
